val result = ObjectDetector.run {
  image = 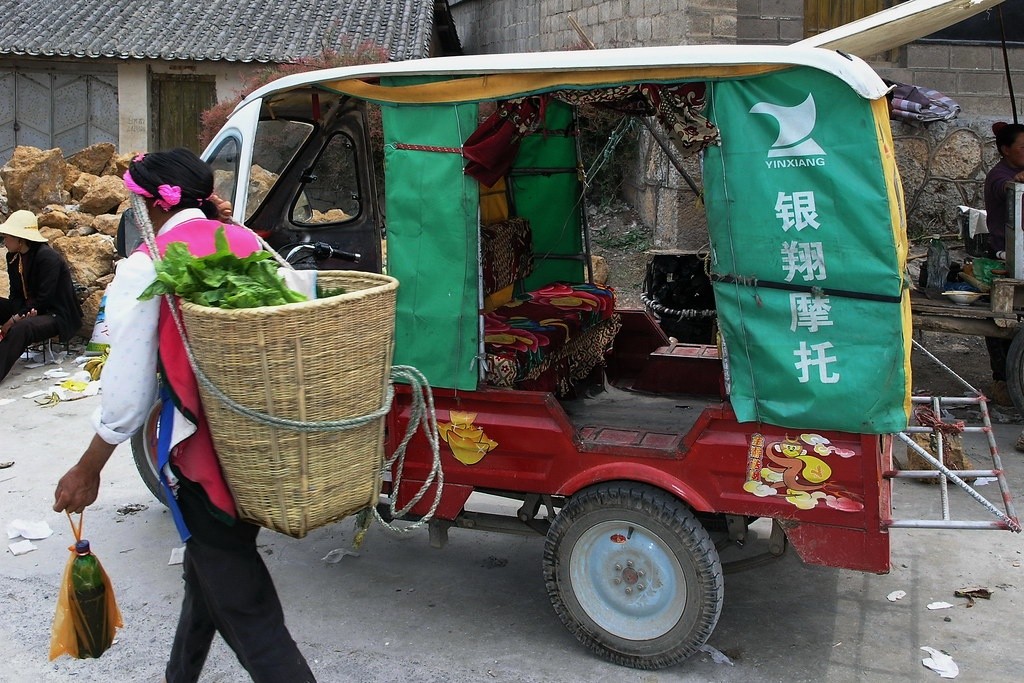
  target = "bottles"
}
[931,234,944,253]
[71,539,110,659]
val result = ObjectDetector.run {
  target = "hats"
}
[0,209,49,242]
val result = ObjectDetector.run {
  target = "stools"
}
[26,335,69,362]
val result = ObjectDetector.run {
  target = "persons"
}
[0,210,84,384]
[985,122,1024,251]
[53,148,319,683]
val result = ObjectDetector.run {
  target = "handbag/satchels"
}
[47,507,124,663]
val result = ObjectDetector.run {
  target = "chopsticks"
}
[941,292,989,295]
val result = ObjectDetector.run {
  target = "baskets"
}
[179,269,399,539]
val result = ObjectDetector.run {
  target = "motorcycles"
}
[129,45,912,670]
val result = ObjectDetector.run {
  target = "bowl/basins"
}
[945,290,980,305]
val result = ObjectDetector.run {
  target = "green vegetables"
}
[136,226,347,310]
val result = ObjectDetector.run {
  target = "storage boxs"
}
[958,213,988,257]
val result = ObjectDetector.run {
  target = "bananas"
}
[84,353,109,381]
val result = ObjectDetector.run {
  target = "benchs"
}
[479,217,622,398]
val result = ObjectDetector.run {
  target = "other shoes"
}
[992,405,1024,422]
